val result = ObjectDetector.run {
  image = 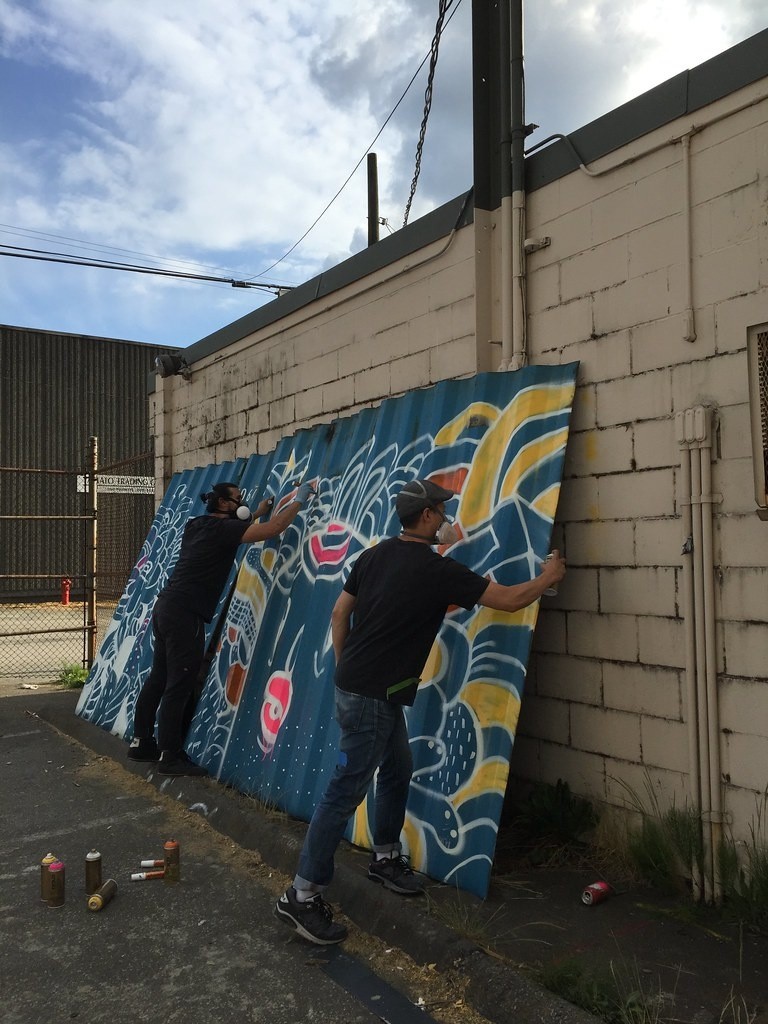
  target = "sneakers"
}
[128,737,160,762]
[157,750,208,776]
[272,888,346,945]
[368,853,423,895]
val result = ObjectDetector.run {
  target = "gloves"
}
[293,481,315,505]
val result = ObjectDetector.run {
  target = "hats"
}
[396,480,455,520]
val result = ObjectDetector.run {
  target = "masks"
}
[226,497,252,523]
[431,508,460,544]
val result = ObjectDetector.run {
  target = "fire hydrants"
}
[60,577,72,605]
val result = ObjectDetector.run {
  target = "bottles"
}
[39,853,66,910]
[84,846,103,896]
[543,551,558,598]
[88,878,118,911]
[164,838,182,883]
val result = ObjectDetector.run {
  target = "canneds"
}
[581,882,608,906]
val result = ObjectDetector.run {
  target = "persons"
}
[271,480,567,948]
[128,476,316,778]
